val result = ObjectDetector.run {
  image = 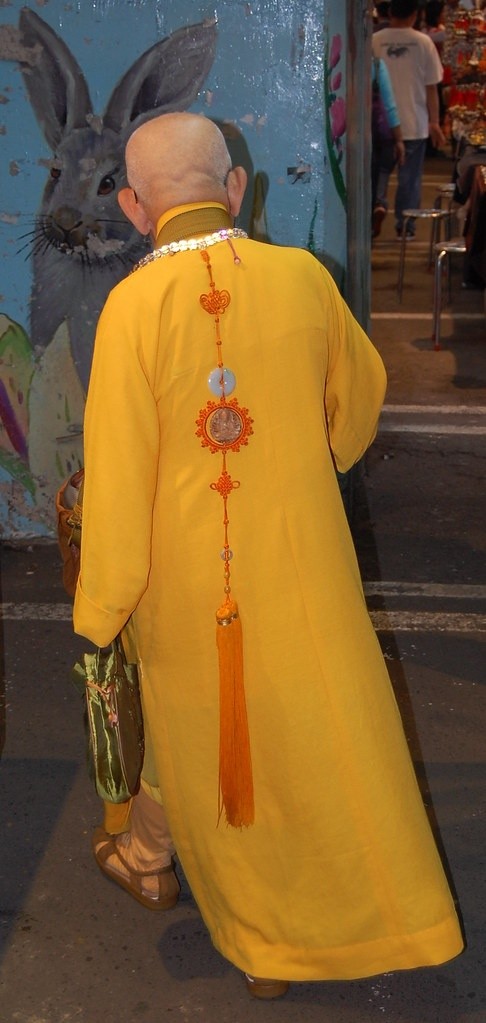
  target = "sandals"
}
[240,970,288,998]
[92,827,180,911]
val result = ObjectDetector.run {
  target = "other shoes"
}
[397,229,416,240]
[372,205,385,236]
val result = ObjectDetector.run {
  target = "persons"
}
[371,0,446,241]
[71,112,469,999]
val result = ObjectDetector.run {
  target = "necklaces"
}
[131,229,248,273]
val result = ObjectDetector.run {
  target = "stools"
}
[432,240,467,351]
[431,184,455,270]
[397,208,452,299]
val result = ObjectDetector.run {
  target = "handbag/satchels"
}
[55,465,81,597]
[372,57,398,173]
[73,640,144,802]
